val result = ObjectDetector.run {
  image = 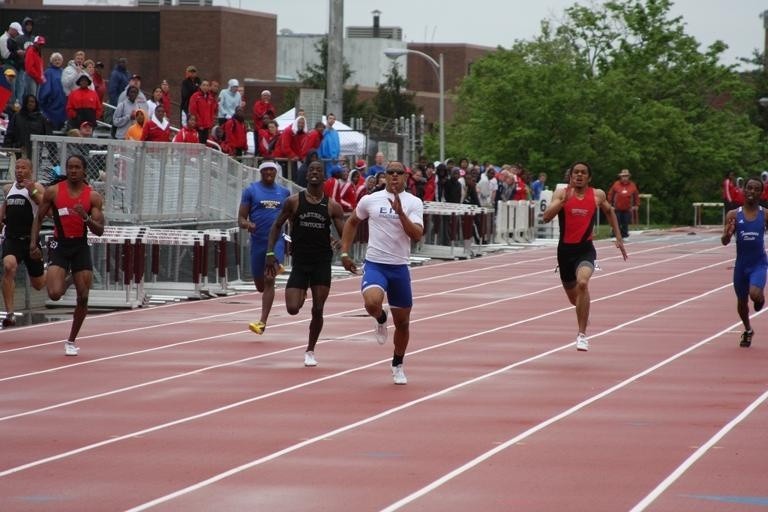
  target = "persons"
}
[341,161,425,385]
[722,169,736,224]
[27,154,104,356]
[1,17,550,245]
[734,177,745,208]
[607,167,641,244]
[239,157,291,333]
[720,178,768,348]
[265,162,347,367]
[543,161,629,351]
[761,171,768,234]
[0,158,48,327]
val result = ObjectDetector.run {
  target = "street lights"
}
[383,46,446,199]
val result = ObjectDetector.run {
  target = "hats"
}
[355,159,366,169]
[33,36,46,45]
[618,169,631,176]
[76,75,91,86]
[330,166,343,175]
[9,22,24,36]
[187,65,196,72]
[4,69,15,77]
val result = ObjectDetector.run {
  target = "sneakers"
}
[623,236,630,241]
[249,322,266,335]
[754,297,765,311]
[577,334,588,351]
[610,237,617,242]
[64,341,78,356]
[304,351,317,367]
[391,363,407,384]
[2,313,17,326]
[376,307,388,344]
[740,329,754,348]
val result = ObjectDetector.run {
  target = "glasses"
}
[386,169,404,175]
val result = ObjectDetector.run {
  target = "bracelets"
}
[84,215,91,222]
[341,253,350,257]
[265,252,274,255]
[31,189,38,195]
